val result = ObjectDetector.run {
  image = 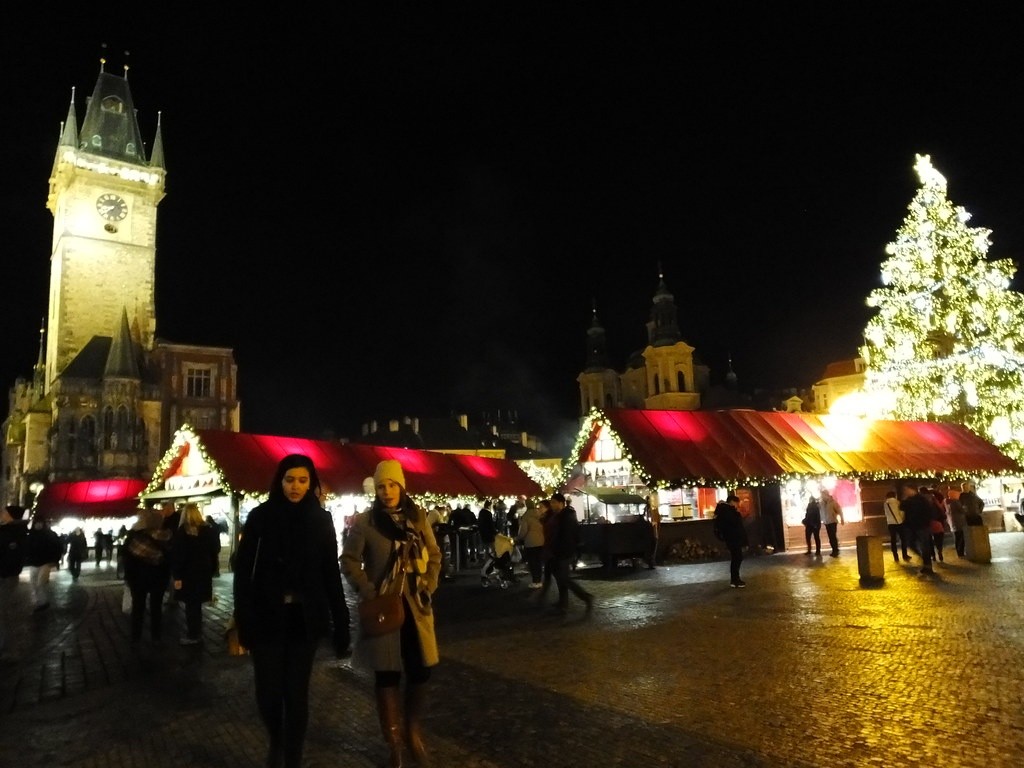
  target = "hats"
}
[373,460,405,490]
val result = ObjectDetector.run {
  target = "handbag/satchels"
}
[122,586,132,614]
[358,593,405,637]
[224,628,247,656]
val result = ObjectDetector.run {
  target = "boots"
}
[376,686,408,768]
[403,680,432,768]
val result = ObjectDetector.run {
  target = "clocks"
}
[95,193,128,221]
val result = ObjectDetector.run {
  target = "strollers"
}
[478,535,522,589]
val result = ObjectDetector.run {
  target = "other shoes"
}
[579,591,594,613]
[730,579,746,588]
[528,583,542,588]
[180,638,200,645]
[807,551,811,554]
[816,551,820,555]
[830,549,839,556]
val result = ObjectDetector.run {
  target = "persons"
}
[802,490,844,558]
[0,506,116,608]
[338,459,442,768]
[230,454,350,768]
[427,494,595,614]
[116,503,220,646]
[713,495,748,588]
[884,482,984,575]
